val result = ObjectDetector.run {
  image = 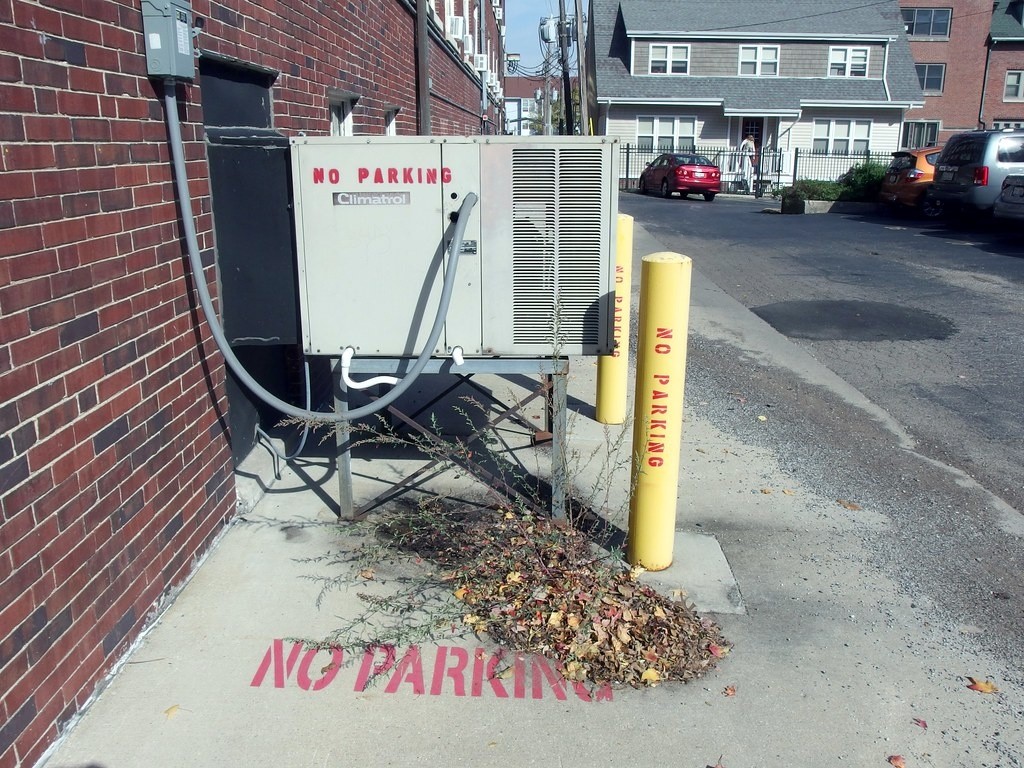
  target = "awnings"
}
[724,97,801,116]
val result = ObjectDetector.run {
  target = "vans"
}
[927,128,1024,220]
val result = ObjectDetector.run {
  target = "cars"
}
[638,153,722,202]
[880,145,945,221]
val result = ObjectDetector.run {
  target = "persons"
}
[740,134,755,169]
